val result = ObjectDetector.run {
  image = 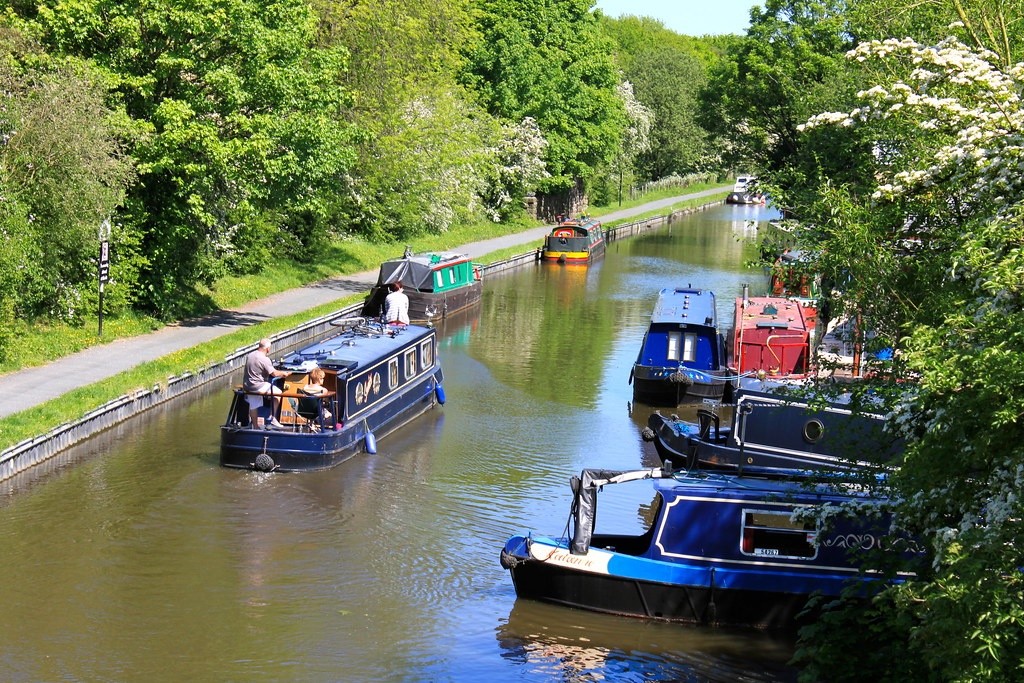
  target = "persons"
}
[243,339,292,430]
[293,369,337,429]
[385,282,410,326]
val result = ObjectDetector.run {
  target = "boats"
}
[634,284,728,405]
[542,215,606,267]
[373,251,485,323]
[220,317,446,472]
[647,373,912,484]
[726,175,766,205]
[499,463,920,629]
[772,248,850,301]
[726,291,819,394]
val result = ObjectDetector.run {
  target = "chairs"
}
[287,396,323,433]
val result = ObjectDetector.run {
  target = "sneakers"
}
[266,414,284,428]
